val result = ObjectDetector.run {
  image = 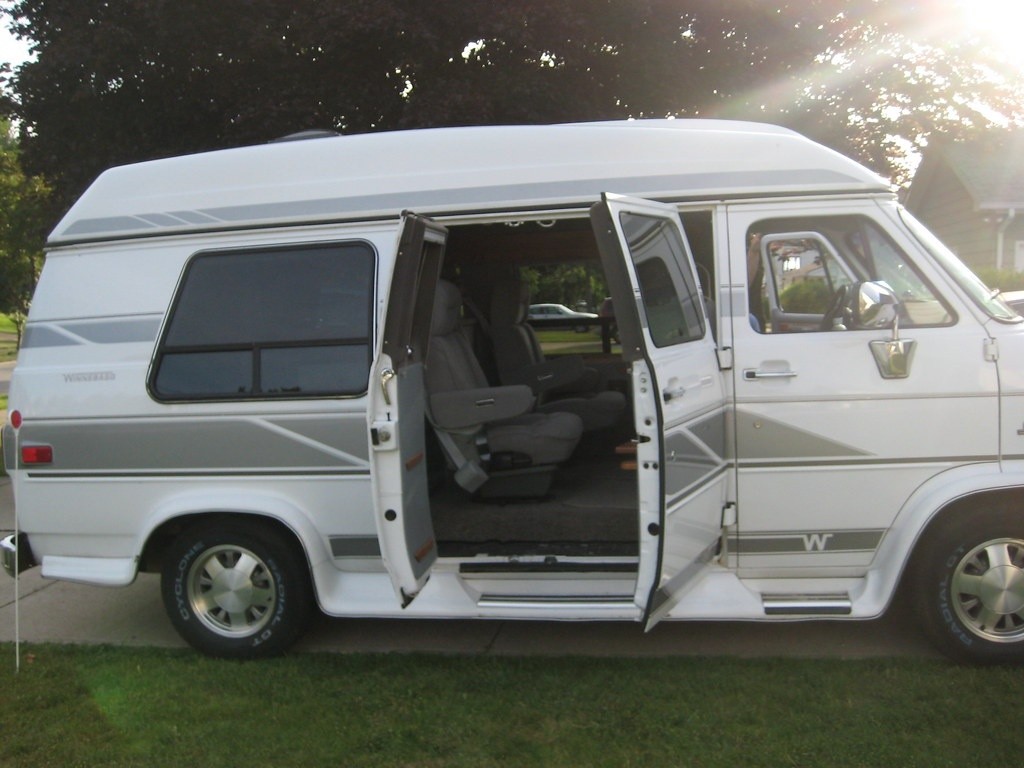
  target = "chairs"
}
[489,277,630,430]
[420,279,585,503]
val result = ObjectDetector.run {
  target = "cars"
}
[526,302,599,333]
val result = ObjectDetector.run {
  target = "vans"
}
[2,119,1023,674]
